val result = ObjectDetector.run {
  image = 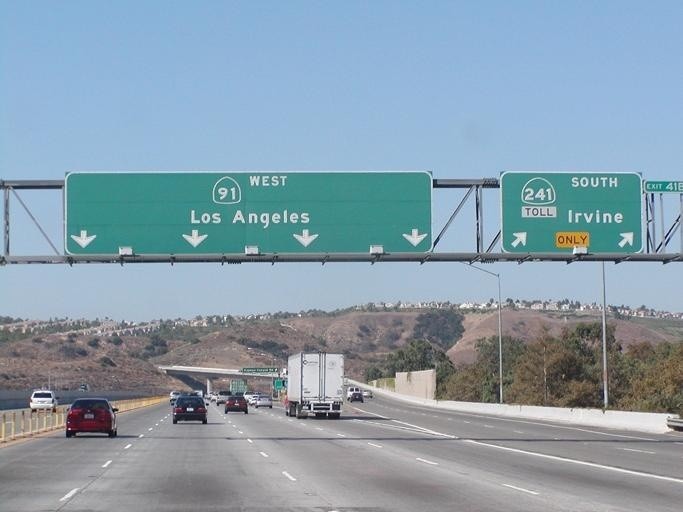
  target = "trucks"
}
[284,351,344,419]
[230,379,248,391]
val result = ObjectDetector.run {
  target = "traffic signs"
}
[501,171,642,254]
[65,172,433,253]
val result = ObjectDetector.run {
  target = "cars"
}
[346,384,372,404]
[169,390,272,424]
[29,390,59,412]
[66,397,119,438]
